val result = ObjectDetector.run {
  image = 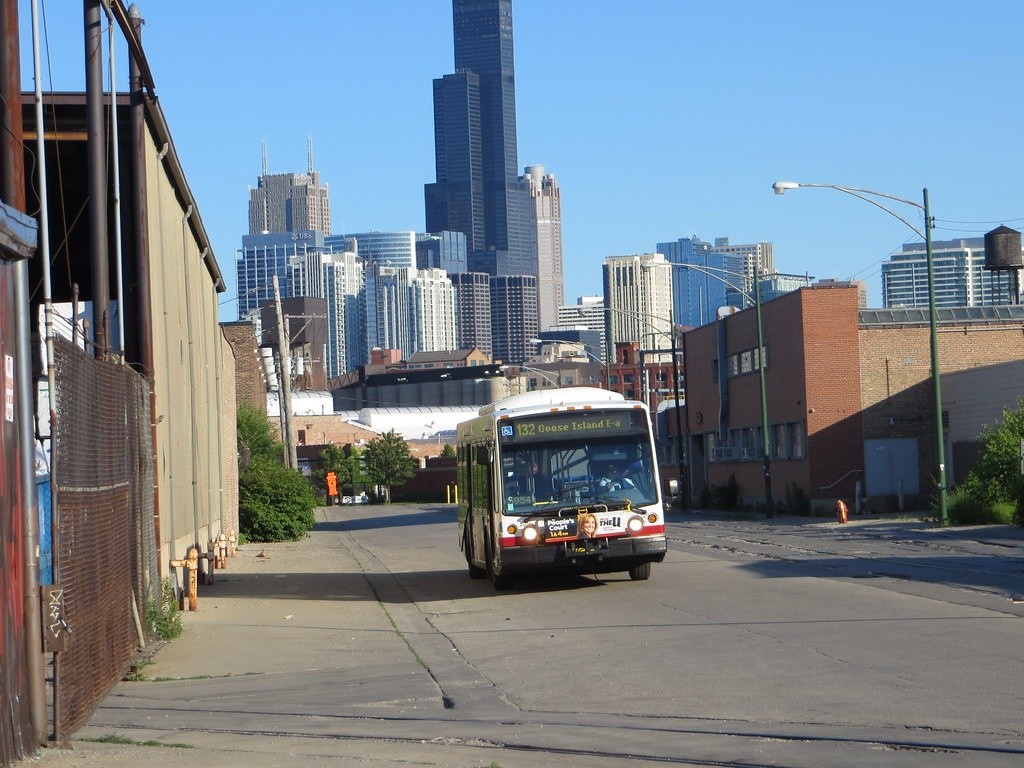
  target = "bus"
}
[456,387,668,592]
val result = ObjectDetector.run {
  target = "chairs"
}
[553,475,587,500]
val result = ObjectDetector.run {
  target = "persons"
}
[597,464,638,495]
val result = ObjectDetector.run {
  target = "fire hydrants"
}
[836,500,849,524]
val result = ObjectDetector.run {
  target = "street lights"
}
[499,365,562,388]
[578,308,687,513]
[475,378,521,394]
[640,262,774,519]
[772,182,951,527]
[529,338,611,391]
